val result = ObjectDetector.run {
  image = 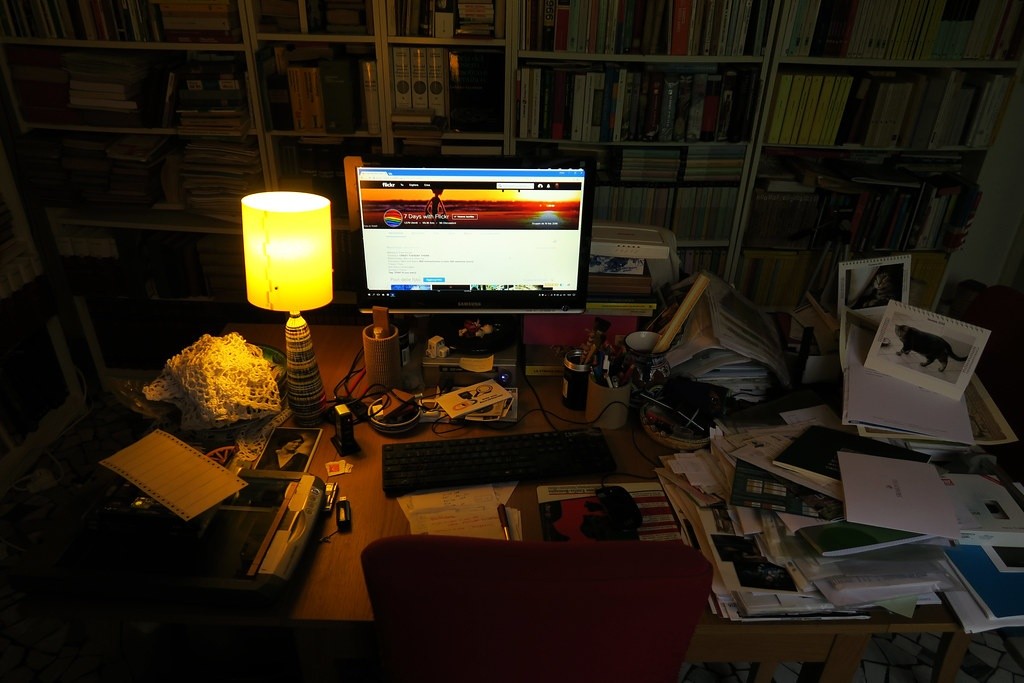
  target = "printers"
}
[60,470,326,617]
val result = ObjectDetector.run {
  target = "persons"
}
[261,433,308,471]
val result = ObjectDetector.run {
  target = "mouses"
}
[596,485,643,531]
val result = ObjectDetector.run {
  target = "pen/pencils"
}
[497,503,512,541]
[591,344,636,389]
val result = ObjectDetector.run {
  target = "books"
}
[0,45,260,300]
[258,0,374,35]
[253,48,504,215]
[519,0,1024,61]
[0,0,242,43]
[737,71,1016,307]
[517,65,761,275]
[387,0,506,38]
[666,275,1024,633]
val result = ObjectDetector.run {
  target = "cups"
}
[586,373,632,429]
[363,325,399,400]
[562,349,593,410]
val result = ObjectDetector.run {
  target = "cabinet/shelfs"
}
[0,0,1024,476]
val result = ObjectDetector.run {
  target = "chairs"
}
[360,534,713,683]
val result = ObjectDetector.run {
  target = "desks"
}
[187,324,977,683]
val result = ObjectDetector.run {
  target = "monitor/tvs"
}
[344,153,596,355]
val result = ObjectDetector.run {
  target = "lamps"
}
[240,191,334,427]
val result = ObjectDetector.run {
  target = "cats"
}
[869,272,895,307]
[894,323,968,372]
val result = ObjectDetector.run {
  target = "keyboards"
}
[382,426,618,493]
